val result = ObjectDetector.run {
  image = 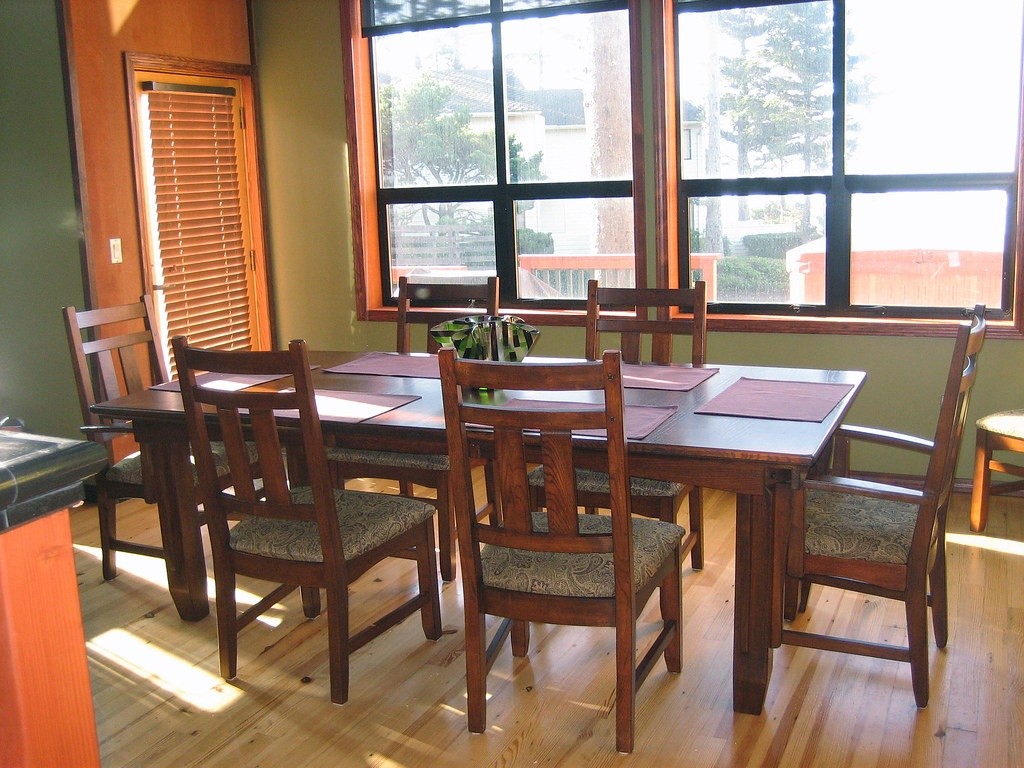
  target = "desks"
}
[89,347,867,715]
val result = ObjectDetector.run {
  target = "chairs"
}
[396,275,504,585]
[436,345,684,755]
[528,278,706,570]
[172,335,443,708]
[765,305,991,709]
[63,295,287,580]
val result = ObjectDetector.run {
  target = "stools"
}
[969,406,1023,533]
